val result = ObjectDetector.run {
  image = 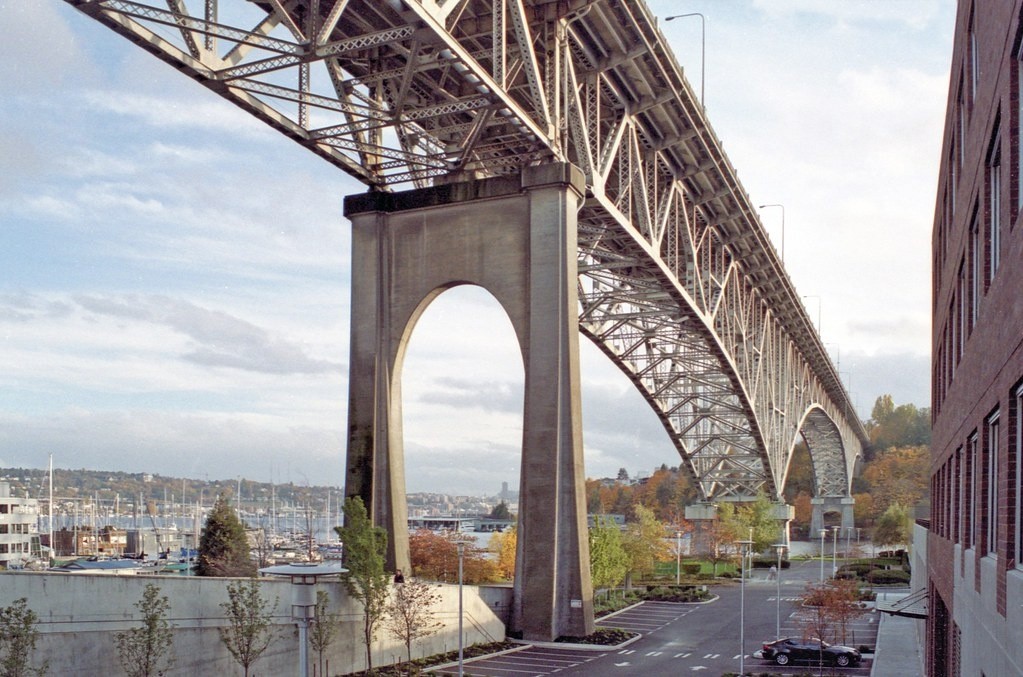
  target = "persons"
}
[394,569,404,583]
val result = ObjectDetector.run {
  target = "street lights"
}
[856,527,862,546]
[747,525,756,580]
[804,295,821,337]
[760,204,786,265]
[448,538,471,677]
[817,528,830,586]
[847,525,854,564]
[771,544,789,640]
[257,563,349,677]
[831,526,841,581]
[665,13,706,109]
[675,530,684,586]
[733,540,757,675]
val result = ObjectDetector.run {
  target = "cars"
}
[761,636,863,667]
